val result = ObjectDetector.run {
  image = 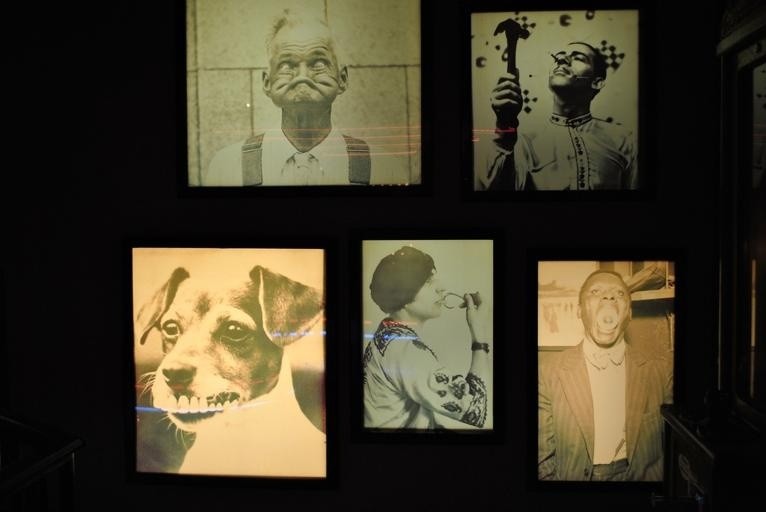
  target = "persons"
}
[360,245,493,430]
[477,40,638,193]
[537,267,674,482]
[200,6,408,185]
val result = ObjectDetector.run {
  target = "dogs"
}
[132,262,329,479]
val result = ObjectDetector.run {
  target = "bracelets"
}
[469,341,490,354]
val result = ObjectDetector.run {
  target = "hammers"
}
[493,19,530,74]
